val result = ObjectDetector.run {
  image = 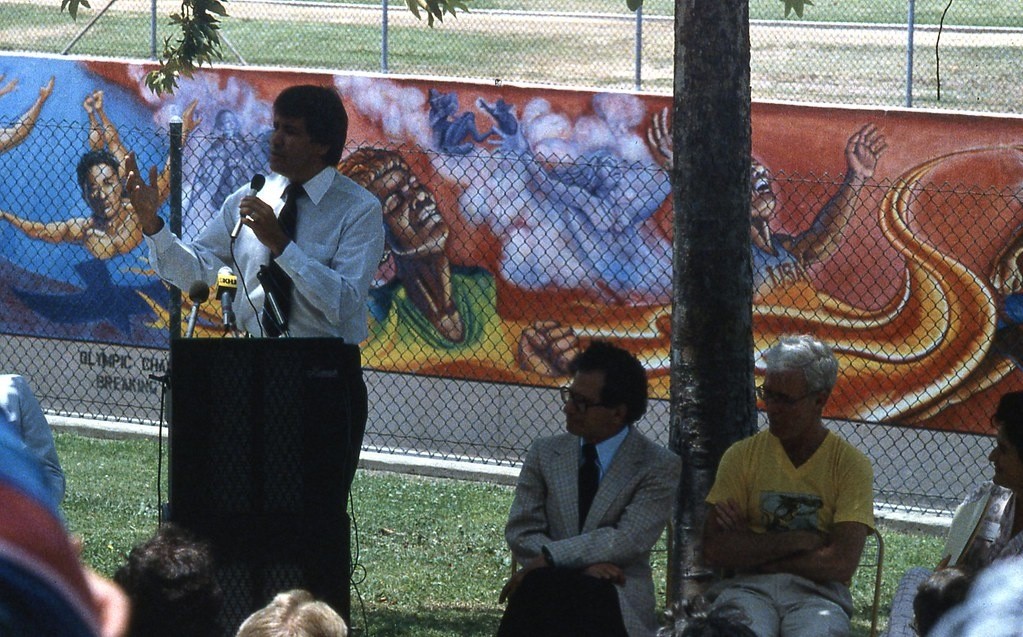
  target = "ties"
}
[577,443,600,536]
[261,183,306,338]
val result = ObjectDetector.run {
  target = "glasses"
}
[561,386,606,414]
[756,378,822,415]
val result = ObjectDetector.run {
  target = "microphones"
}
[215,266,236,328]
[256,269,287,331]
[186,280,209,337]
[230,174,266,239]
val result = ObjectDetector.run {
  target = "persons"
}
[911,389,1023,637]
[495,342,683,637]
[702,334,876,637]
[0,373,130,637]
[236,589,347,637]
[115,522,225,637]
[125,84,384,637]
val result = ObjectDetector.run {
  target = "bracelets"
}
[544,549,553,564]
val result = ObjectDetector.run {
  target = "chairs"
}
[722,527,884,637]
[512,516,674,637]
